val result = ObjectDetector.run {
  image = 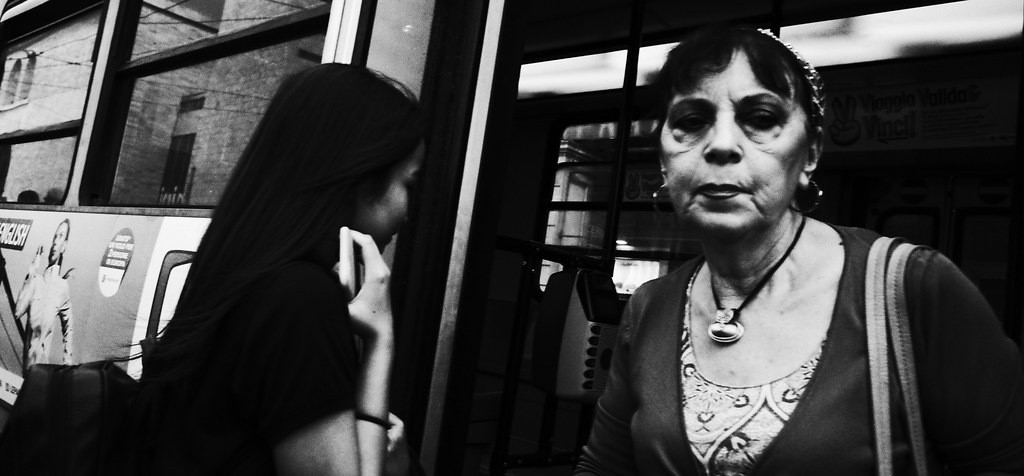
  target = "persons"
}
[107,62,429,476]
[13,218,75,378]
[571,26,1024,476]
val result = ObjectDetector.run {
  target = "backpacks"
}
[0,357,139,476]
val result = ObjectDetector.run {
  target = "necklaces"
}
[708,215,806,345]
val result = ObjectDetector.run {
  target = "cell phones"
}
[338,226,361,301]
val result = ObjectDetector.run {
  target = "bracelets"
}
[355,412,392,431]
